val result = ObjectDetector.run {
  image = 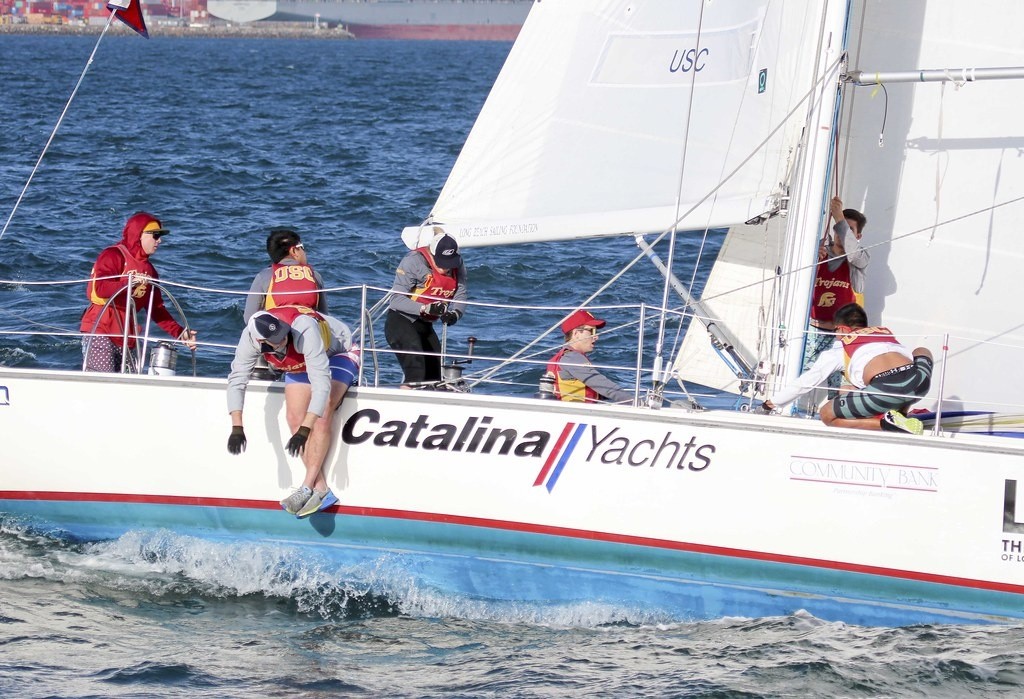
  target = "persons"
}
[803,197,871,397]
[81,212,197,374]
[384,232,466,389]
[753,303,933,435]
[227,306,360,520]
[242,230,329,383]
[539,310,648,405]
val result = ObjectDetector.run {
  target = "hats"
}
[430,233,463,267]
[142,221,169,235]
[562,310,606,333]
[248,311,290,343]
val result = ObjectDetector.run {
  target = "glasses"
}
[295,243,304,250]
[575,328,597,336]
[143,231,161,240]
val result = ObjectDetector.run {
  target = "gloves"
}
[750,400,771,415]
[425,302,448,316]
[441,309,461,326]
[227,425,247,454]
[285,426,310,457]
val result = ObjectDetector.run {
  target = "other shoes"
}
[881,410,924,436]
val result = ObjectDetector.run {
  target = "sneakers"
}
[296,487,339,519]
[280,485,313,514]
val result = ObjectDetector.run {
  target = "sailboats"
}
[0,1,1024,635]
